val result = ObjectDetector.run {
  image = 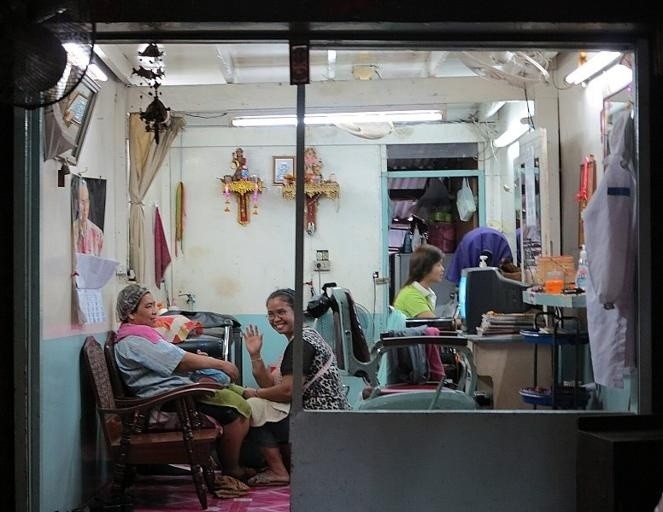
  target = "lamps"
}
[231,110,445,127]
[490,122,534,151]
[563,51,620,88]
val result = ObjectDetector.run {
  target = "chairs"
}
[324,285,481,412]
[79,335,221,510]
[104,329,225,434]
[387,304,461,379]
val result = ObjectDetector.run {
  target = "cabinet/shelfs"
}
[519,311,591,411]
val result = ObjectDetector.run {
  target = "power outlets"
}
[372,271,378,278]
[313,260,330,271]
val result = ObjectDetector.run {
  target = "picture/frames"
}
[273,156,294,183]
[577,161,597,250]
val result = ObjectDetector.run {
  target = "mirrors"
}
[600,84,635,170]
[64,92,89,130]
[51,65,102,165]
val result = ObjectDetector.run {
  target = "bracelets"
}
[251,358,261,361]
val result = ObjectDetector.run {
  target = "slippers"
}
[247,473,288,486]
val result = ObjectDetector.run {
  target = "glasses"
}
[268,310,289,319]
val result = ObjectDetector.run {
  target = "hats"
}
[117,285,149,322]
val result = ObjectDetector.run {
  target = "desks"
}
[457,327,559,414]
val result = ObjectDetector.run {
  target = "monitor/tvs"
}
[458,267,531,333]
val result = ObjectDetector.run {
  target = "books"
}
[477,311,540,336]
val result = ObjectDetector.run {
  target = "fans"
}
[314,303,375,358]
[453,49,550,88]
[0,0,97,110]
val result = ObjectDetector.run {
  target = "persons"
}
[73,180,102,254]
[243,289,352,485]
[114,285,256,481]
[389,244,463,386]
